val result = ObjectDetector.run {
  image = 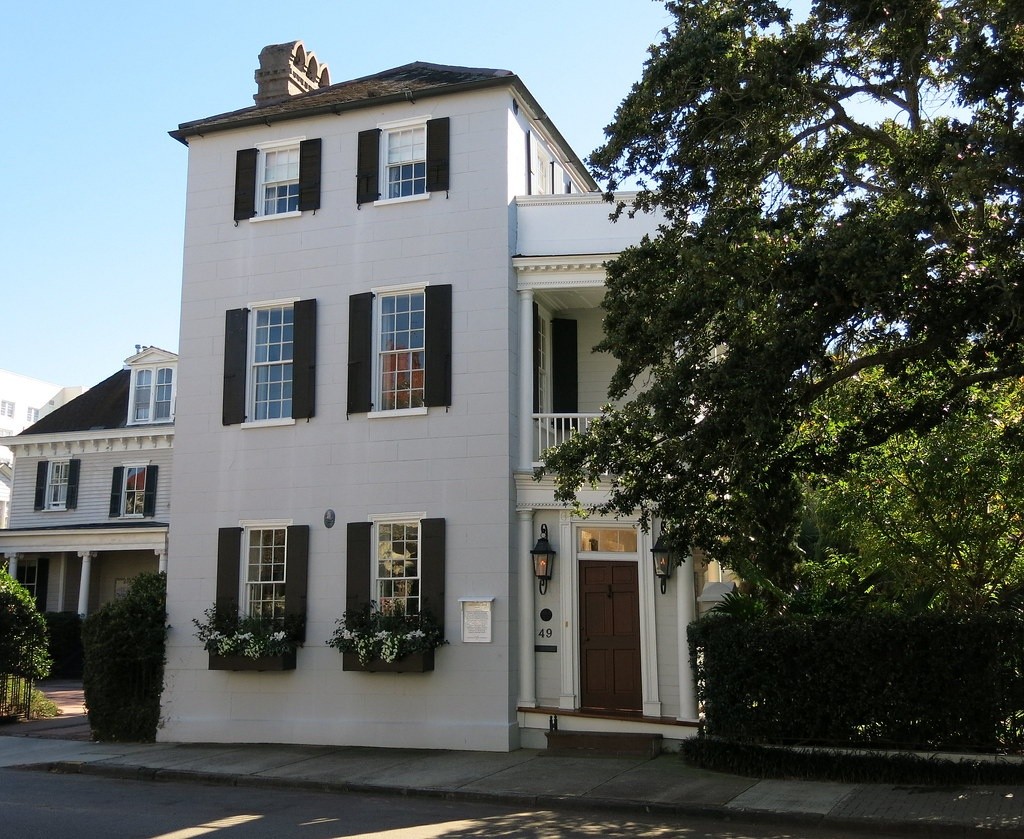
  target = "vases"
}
[209,651,297,671]
[342,652,435,673]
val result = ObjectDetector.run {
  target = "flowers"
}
[325,598,450,667]
[191,599,306,660]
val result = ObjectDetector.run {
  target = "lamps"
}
[651,518,679,594]
[530,523,556,595]
